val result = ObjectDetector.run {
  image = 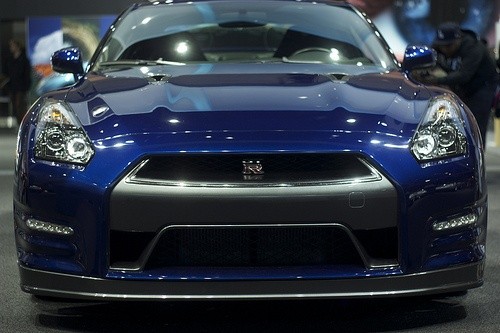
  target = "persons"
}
[413,24,500,148]
[0,38,34,128]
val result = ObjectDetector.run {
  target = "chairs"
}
[272,25,354,63]
[124,29,209,65]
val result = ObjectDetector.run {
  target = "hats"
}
[433,20,463,46]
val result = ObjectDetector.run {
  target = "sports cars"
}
[13,1,486,316]
